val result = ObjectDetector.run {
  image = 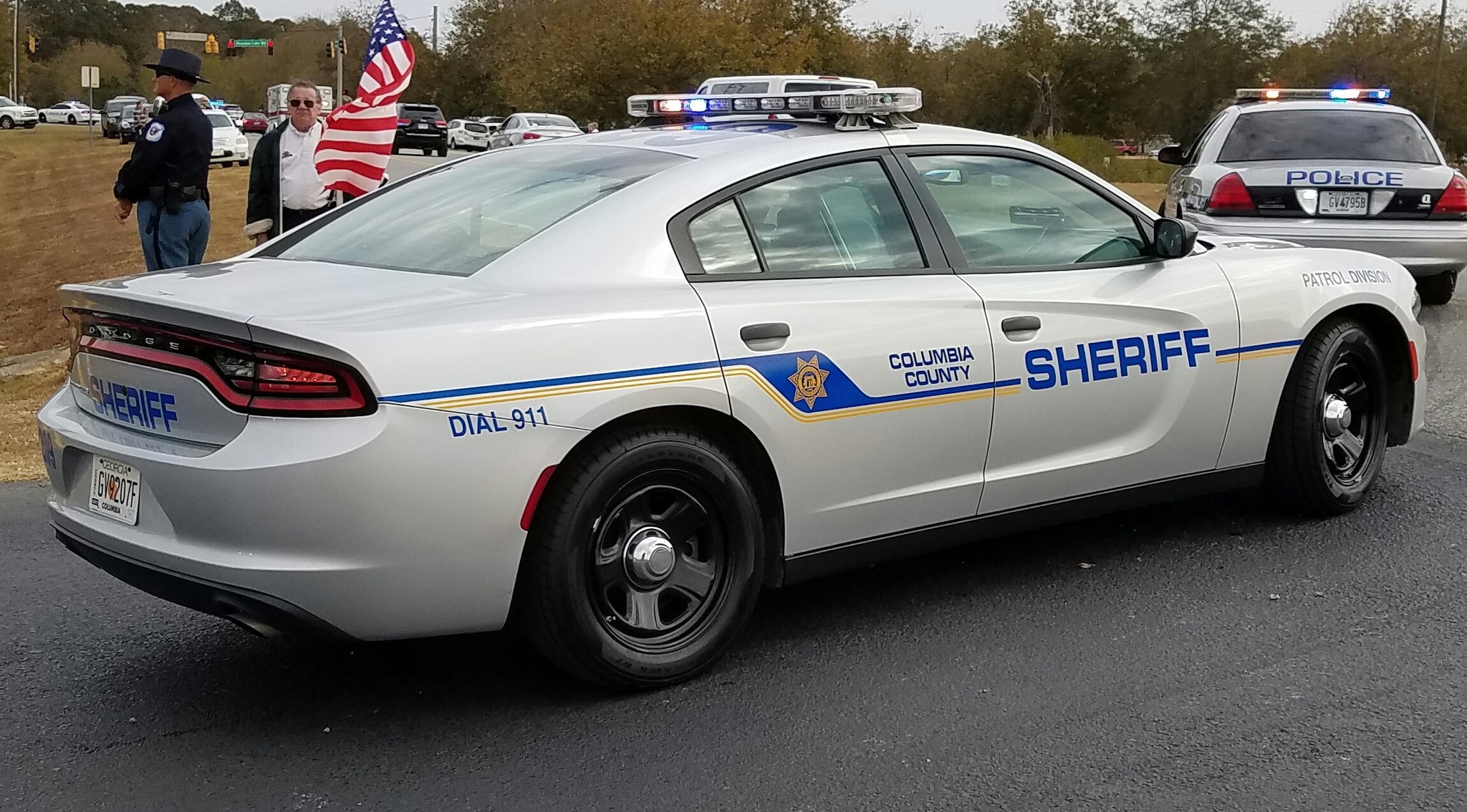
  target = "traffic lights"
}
[326,41,335,58]
[268,40,273,55]
[227,39,236,57]
[339,37,348,55]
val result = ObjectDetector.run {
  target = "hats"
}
[142,48,212,83]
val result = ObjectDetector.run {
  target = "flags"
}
[315,0,417,199]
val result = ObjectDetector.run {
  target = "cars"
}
[1,95,581,167]
[1156,88,1467,305]
[1150,144,1178,156]
[34,87,1425,682]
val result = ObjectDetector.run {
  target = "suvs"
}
[1109,138,1137,157]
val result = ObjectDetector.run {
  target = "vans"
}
[692,75,877,92]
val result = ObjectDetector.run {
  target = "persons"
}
[246,82,335,247]
[142,108,150,125]
[588,121,599,134]
[113,48,211,272]
[133,101,147,139]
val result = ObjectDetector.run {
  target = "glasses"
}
[156,70,173,78]
[287,99,318,108]
